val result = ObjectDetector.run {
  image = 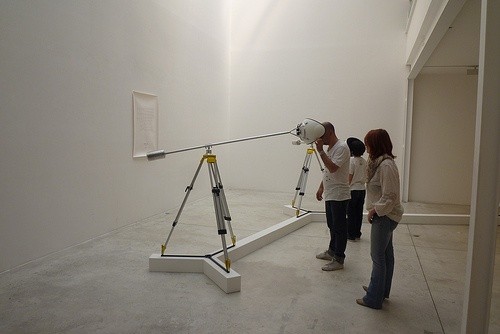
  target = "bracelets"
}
[369,210,373,218]
[319,151,325,155]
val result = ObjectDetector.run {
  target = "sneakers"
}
[321,259,344,271]
[316,250,335,261]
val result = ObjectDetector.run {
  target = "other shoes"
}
[356,298,367,306]
[363,286,369,292]
[347,233,361,240]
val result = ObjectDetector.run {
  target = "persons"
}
[347,137,368,240]
[356,129,404,310]
[314,122,351,271]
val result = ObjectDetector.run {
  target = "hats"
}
[347,137,366,155]
